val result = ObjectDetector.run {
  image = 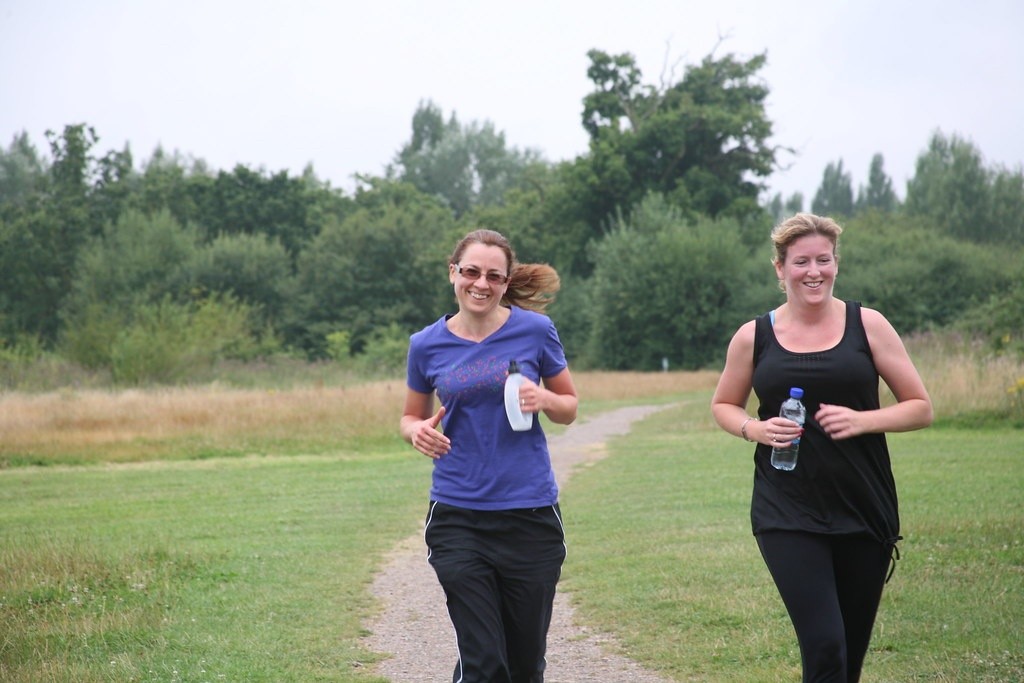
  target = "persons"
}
[710,213,933,682]
[401,229,576,682]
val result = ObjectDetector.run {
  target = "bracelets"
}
[740,416,758,443]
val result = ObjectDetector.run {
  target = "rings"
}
[522,397,525,405]
[772,433,776,442]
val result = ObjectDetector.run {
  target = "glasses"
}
[454,263,509,285]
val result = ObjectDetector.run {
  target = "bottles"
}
[770,387,806,472]
[504,357,533,431]
